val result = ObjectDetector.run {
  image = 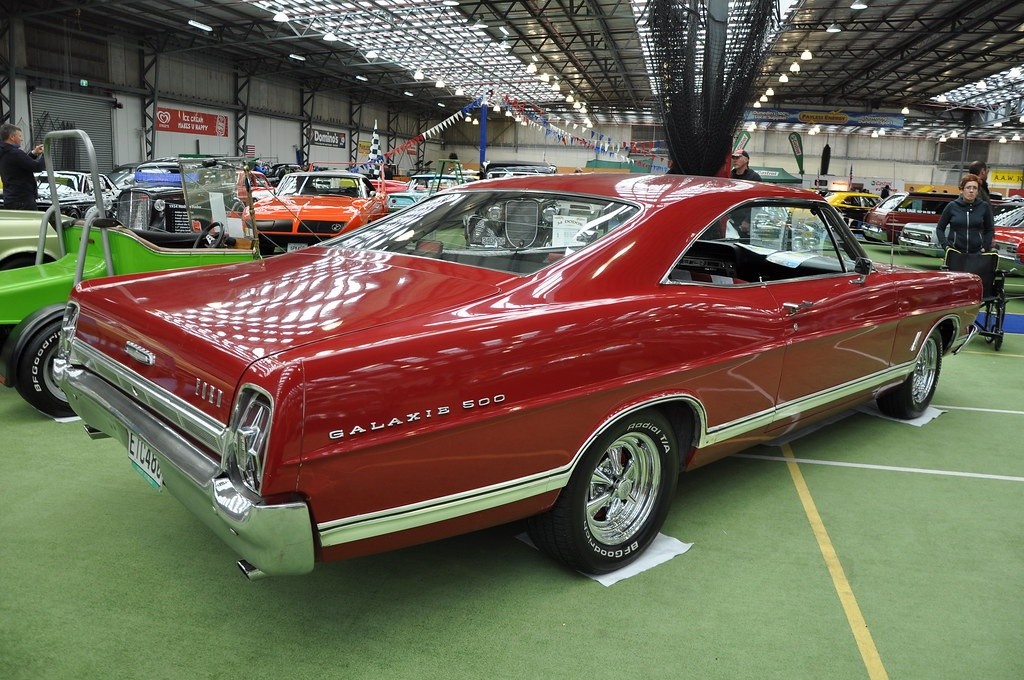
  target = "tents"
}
[751,166,802,184]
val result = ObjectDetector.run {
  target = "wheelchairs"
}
[940,244,1019,352]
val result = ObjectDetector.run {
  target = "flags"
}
[368,125,384,181]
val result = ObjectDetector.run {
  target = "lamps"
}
[365,49,379,58]
[498,36,513,49]
[272,5,290,22]
[442,0,462,7]
[434,78,445,88]
[455,85,528,126]
[356,75,369,82]
[745,0,1024,143]
[413,67,424,80]
[526,61,536,74]
[287,54,307,61]
[473,17,489,29]
[188,19,214,32]
[540,72,588,114]
[323,26,338,41]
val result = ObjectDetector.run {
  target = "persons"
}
[881,185,889,199]
[852,186,869,193]
[936,174,995,253]
[0,124,45,211]
[969,160,990,202]
[729,150,762,238]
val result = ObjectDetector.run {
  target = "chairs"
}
[679,238,769,282]
[299,186,319,196]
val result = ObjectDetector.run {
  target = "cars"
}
[27,159,299,253]
[858,191,1018,244]
[788,192,881,224]
[62,173,988,584]
[242,171,387,256]
[370,162,561,250]
[991,207,1024,266]
[898,207,1017,259]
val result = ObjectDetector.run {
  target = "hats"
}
[731,149,749,161]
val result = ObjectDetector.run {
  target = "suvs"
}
[0,131,258,415]
